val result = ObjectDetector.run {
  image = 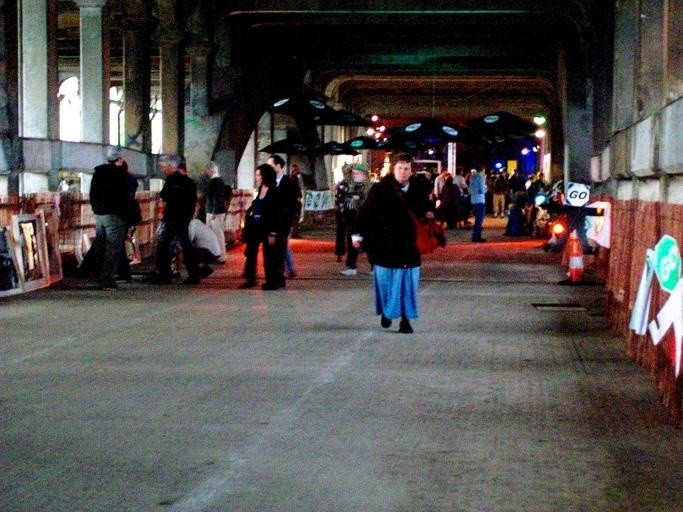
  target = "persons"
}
[86,144,233,291]
[239,154,316,291]
[348,150,437,334]
[335,161,565,275]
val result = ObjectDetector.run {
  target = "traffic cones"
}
[557,229,592,285]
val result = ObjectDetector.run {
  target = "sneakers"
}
[399,320,413,333]
[381,314,391,328]
[340,266,357,275]
[336,256,344,263]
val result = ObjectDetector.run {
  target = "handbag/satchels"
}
[416,223,446,255]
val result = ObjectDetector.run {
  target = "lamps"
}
[533,113,546,126]
[534,129,546,139]
[366,114,386,138]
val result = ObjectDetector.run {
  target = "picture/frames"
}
[0,226,25,298]
[12,211,51,293]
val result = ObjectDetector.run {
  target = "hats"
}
[107,147,121,161]
